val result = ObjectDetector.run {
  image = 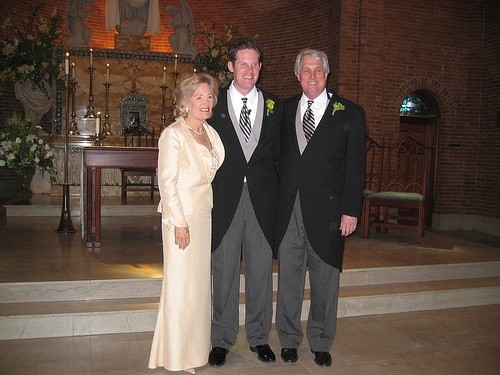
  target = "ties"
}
[303,100,316,145]
[239,97,251,142]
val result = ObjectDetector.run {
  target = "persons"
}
[155,40,281,366]
[166,5,197,51]
[275,48,365,368]
[147,73,225,375]
[68,0,95,42]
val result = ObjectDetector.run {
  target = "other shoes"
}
[183,368,196,375]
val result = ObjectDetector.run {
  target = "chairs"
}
[357,133,431,243]
[121,125,157,202]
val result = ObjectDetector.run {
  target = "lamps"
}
[65,52,69,75]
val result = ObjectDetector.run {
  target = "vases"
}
[0,166,36,203]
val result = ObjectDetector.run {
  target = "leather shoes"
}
[311,348,332,366]
[209,347,230,366]
[250,344,276,362]
[281,348,298,362]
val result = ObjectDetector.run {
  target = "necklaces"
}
[187,124,206,135]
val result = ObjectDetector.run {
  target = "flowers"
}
[266,99,275,117]
[332,101,345,115]
[0,113,60,185]
[0,5,63,103]
[190,21,256,85]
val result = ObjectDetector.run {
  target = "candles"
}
[90,48,93,66]
[106,64,110,83]
[163,66,166,84]
[95,116,100,133]
[72,62,76,82]
[174,54,178,73]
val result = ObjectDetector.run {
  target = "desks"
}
[80,145,159,246]
